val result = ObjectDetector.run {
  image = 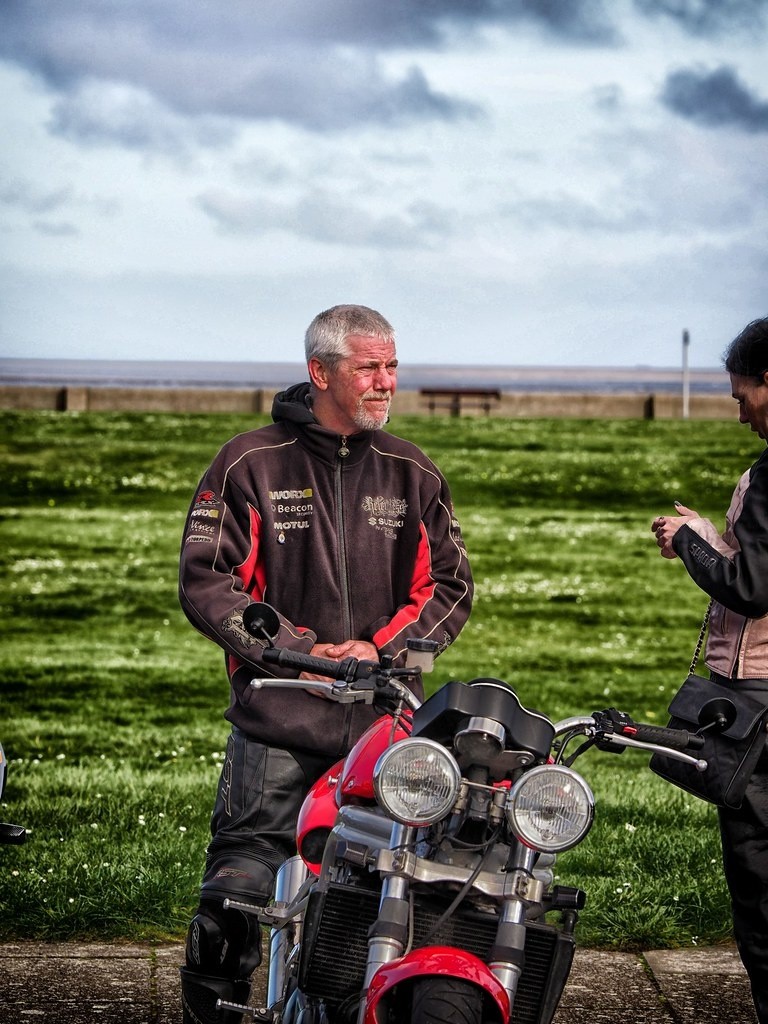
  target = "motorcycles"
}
[215,602,708,1024]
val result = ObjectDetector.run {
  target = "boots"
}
[179,967,254,1024]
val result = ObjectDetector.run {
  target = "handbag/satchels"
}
[649,674,768,810]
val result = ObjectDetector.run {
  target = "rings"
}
[656,526,662,535]
[658,516,665,525]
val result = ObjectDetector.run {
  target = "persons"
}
[178,305,473,1024]
[650,316,768,1024]
